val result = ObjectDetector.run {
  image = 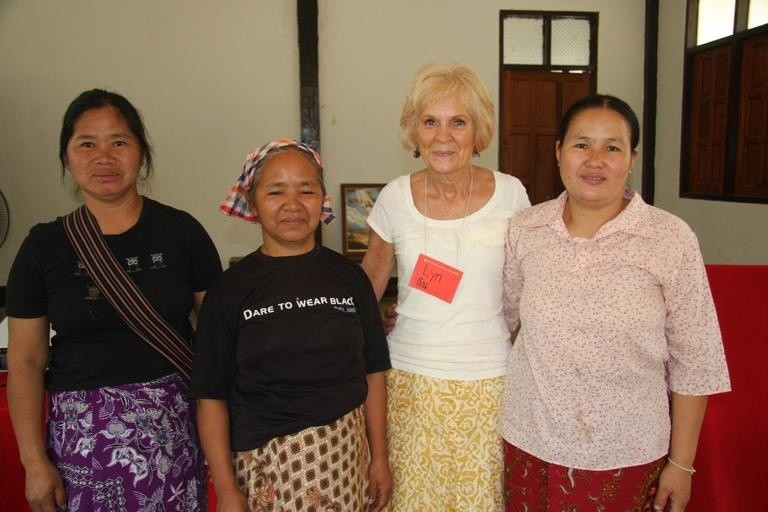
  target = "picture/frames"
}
[337,182,395,261]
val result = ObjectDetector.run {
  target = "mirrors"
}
[675,0,768,206]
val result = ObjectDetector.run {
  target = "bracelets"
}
[666,455,696,475]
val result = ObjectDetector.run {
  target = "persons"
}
[4,86,224,512]
[188,137,395,512]
[500,93,732,512]
[357,63,533,512]
